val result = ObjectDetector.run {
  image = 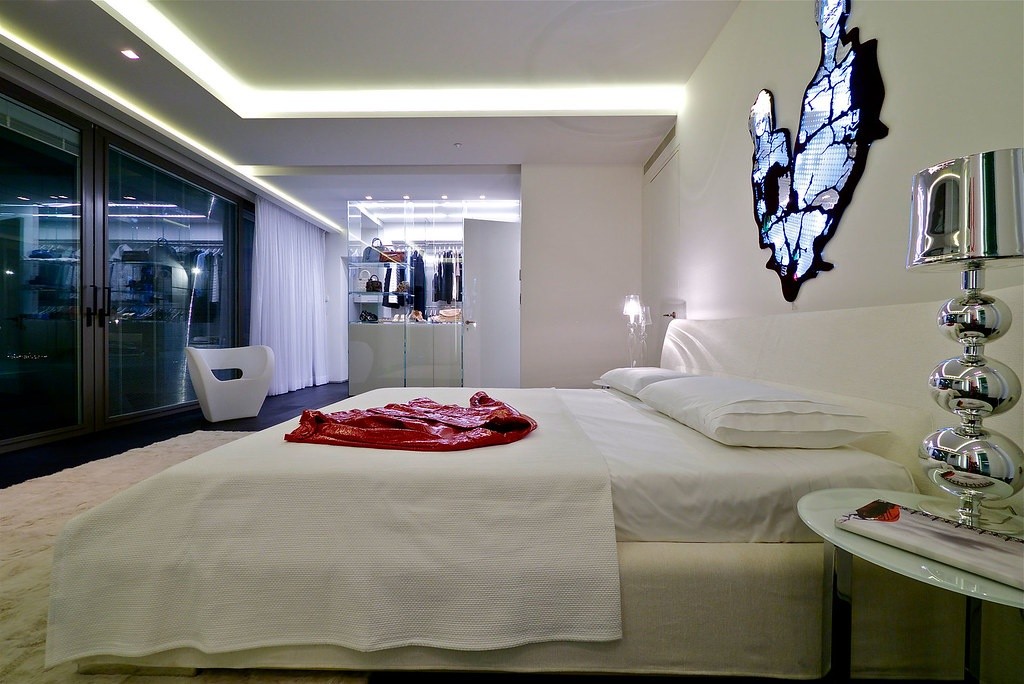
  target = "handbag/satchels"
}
[354,269,373,291]
[365,274,381,292]
[362,237,388,262]
[379,248,406,262]
[438,309,461,322]
[149,237,177,262]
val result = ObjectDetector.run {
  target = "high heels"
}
[411,310,426,322]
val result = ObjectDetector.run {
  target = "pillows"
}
[599,367,694,398]
[637,377,892,449]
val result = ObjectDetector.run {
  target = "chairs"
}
[185,346,275,423]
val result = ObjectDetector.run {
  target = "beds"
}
[44,285,1023,677]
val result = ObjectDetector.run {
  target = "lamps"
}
[906,149,1024,535]
[624,296,653,369]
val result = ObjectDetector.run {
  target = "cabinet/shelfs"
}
[341,239,463,396]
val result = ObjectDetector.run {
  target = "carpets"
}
[0,429,370,684]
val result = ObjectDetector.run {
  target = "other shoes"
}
[109,304,190,322]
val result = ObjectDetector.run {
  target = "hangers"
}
[436,247,464,253]
[175,244,223,252]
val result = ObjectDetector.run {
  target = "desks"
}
[797,491,1024,684]
